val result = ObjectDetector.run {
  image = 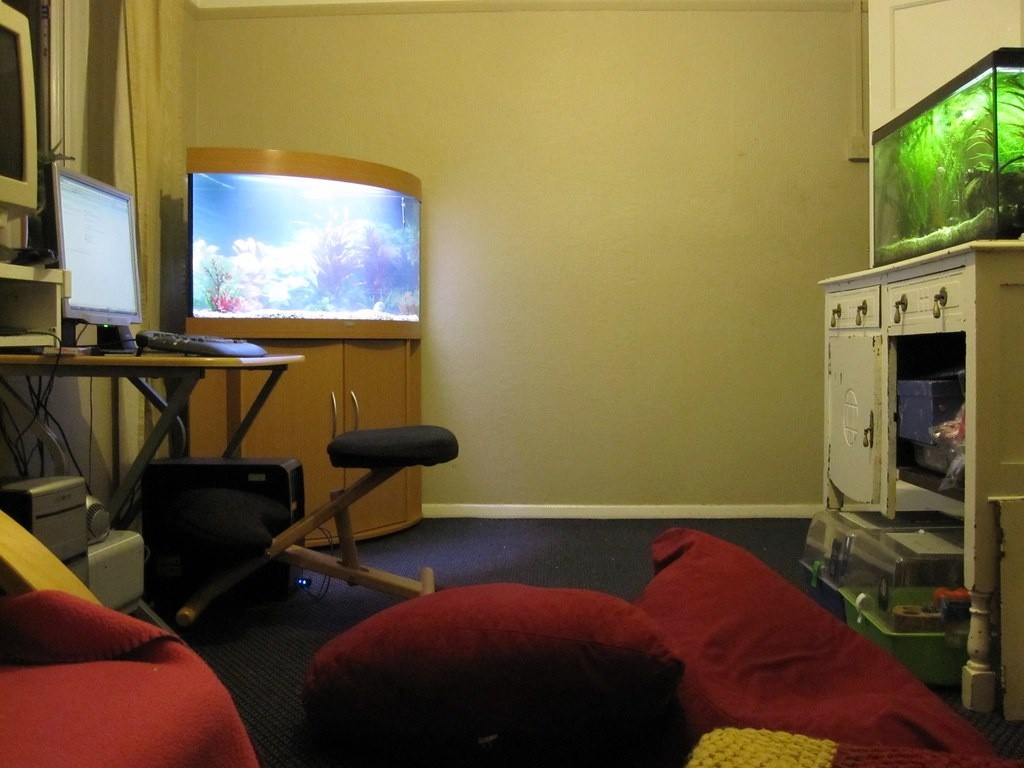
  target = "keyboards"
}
[137,329,268,358]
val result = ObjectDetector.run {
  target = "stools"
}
[174,425,458,628]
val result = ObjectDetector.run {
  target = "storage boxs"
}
[836,527,965,688]
[185,145,423,339]
[897,379,964,445]
[797,508,965,612]
[870,49,1024,269]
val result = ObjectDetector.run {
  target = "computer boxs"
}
[0,475,89,589]
[140,458,305,609]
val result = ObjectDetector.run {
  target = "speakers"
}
[86,494,109,544]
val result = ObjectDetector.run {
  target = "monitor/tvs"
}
[0,0,38,262]
[46,161,143,355]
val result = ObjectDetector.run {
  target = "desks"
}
[0,350,310,644]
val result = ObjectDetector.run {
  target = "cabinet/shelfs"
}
[816,238,1024,714]
[186,339,425,548]
